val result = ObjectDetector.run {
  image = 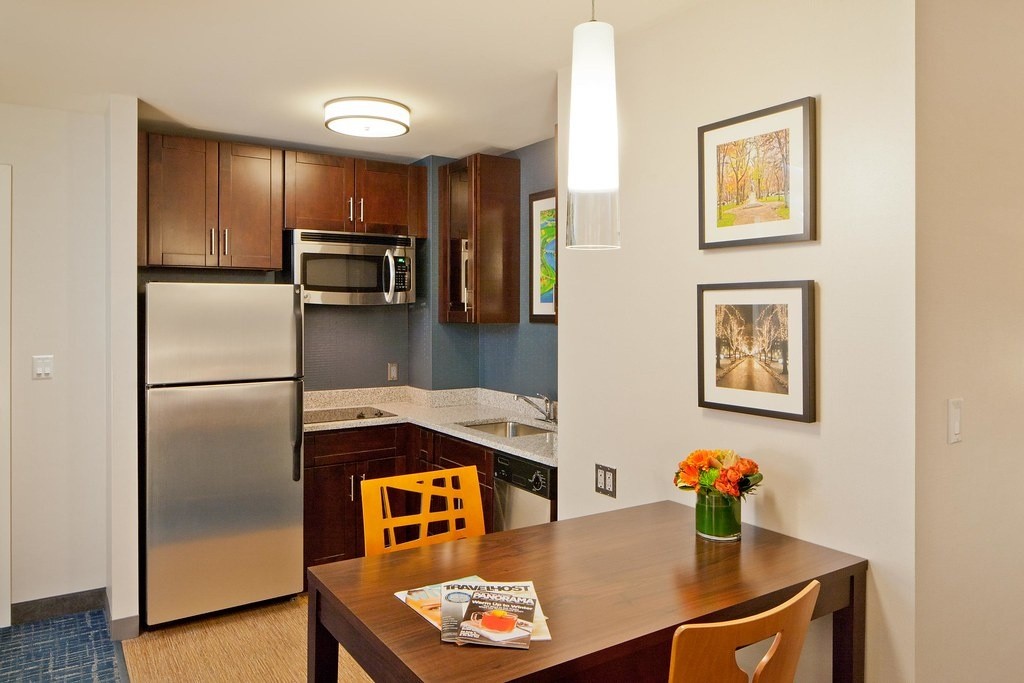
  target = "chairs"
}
[667,579,820,683]
[359,465,486,556]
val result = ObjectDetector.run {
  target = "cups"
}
[471,610,518,633]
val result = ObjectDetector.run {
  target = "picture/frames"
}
[699,97,817,251]
[697,279,816,423]
[528,189,556,325]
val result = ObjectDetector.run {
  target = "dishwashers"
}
[490,452,557,533]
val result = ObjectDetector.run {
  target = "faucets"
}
[513,392,555,422]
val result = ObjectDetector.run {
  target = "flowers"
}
[674,449,763,505]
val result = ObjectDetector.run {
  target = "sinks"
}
[452,418,558,439]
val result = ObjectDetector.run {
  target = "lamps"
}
[323,97,411,138]
[565,0,621,250]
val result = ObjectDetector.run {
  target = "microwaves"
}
[276,229,417,306]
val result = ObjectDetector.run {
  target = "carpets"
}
[0,608,130,683]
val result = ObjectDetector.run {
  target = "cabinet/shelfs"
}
[283,151,428,239]
[146,133,283,272]
[304,423,408,591]
[407,424,493,541]
[439,153,521,325]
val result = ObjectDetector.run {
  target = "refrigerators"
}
[137,282,306,629]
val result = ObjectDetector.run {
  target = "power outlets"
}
[388,362,398,381]
[595,464,617,499]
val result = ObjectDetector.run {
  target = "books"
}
[393,574,552,647]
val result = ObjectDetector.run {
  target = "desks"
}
[306,498,867,683]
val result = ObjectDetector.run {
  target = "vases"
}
[695,491,742,542]
